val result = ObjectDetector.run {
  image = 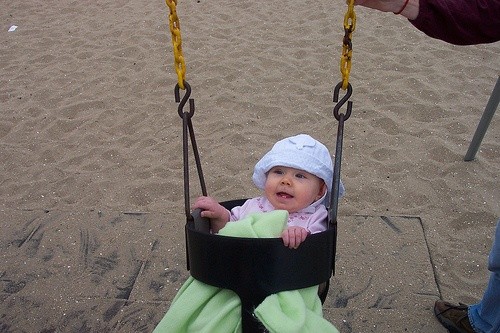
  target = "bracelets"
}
[392,0,409,15]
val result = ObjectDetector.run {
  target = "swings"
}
[164,0,358,333]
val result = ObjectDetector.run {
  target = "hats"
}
[253,134,345,200]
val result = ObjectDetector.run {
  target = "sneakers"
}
[434,300,476,333]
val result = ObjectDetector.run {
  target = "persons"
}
[357,0,500,333]
[152,134,345,333]
